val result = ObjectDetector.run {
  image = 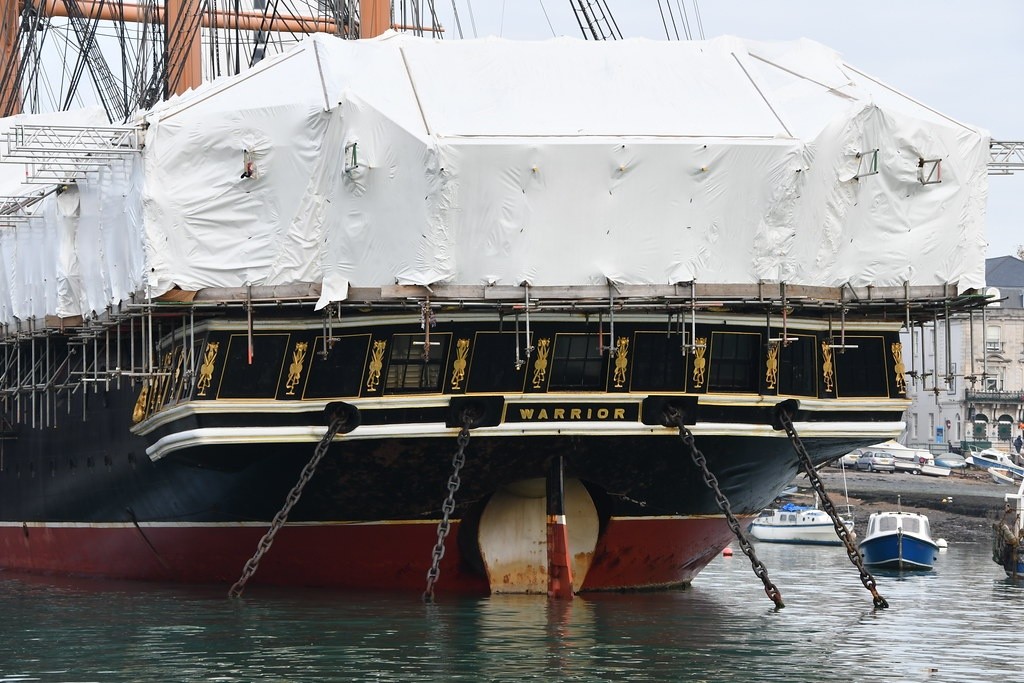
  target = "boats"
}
[987,466,1024,486]
[0,0,1002,595]
[934,453,967,468]
[965,456,979,471]
[859,439,932,458]
[859,511,937,573]
[967,444,1024,477]
[991,479,1023,580]
[750,505,853,545]
[895,458,951,476]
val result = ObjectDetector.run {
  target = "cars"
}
[828,450,863,468]
[853,451,895,473]
